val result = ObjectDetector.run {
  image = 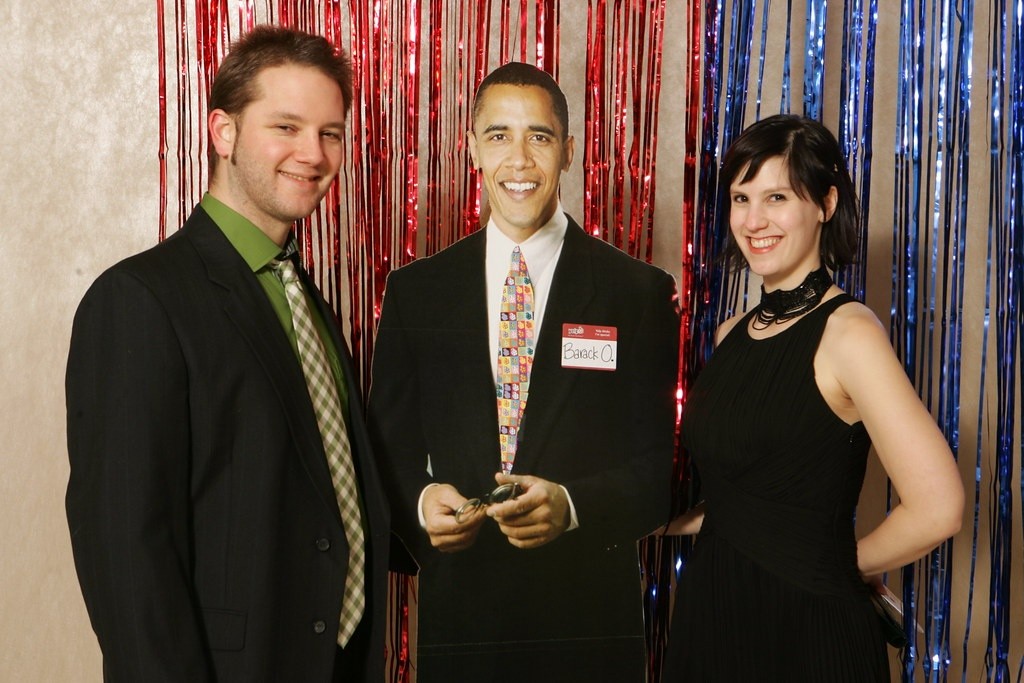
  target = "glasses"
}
[455,482,523,524]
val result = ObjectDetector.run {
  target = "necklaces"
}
[751,263,833,331]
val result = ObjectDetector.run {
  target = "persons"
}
[63,25,391,682]
[658,112,966,683]
[357,60,681,682]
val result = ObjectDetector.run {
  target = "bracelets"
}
[658,522,671,537]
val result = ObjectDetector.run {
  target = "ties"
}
[267,257,365,649]
[496,246,534,476]
